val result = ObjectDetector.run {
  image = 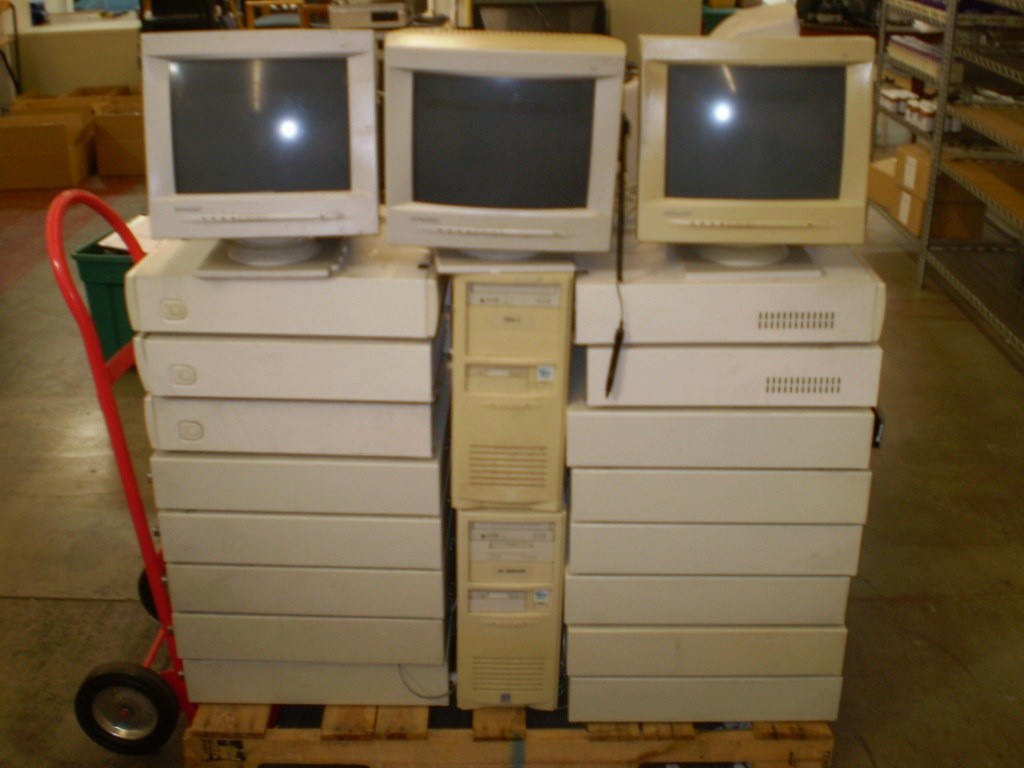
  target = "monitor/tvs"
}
[142,26,377,278]
[637,32,876,268]
[385,30,628,274]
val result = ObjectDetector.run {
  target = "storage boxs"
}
[1,94,146,189]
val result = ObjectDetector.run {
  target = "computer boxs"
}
[448,271,574,511]
[456,511,568,711]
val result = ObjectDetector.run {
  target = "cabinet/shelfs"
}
[869,0,1024,372]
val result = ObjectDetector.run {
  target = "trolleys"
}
[43,185,288,753]
